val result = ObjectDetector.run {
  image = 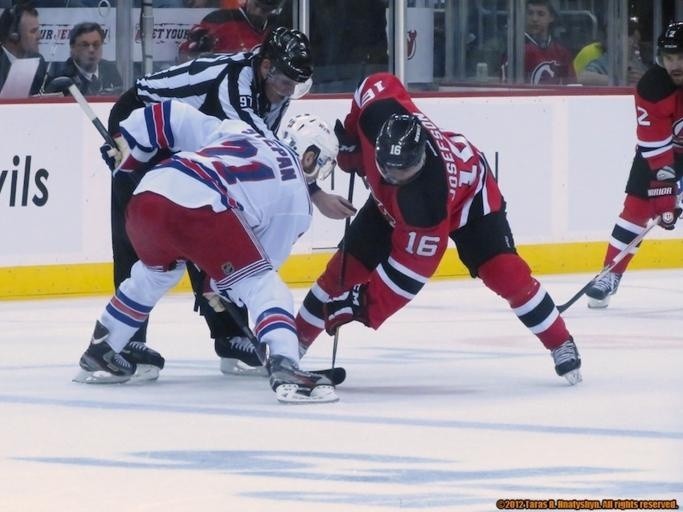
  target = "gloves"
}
[100,133,144,180]
[202,275,230,313]
[649,181,683,230]
[322,283,369,336]
[335,119,366,177]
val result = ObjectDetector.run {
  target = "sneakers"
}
[586,271,621,300]
[215,335,266,365]
[267,356,335,392]
[120,340,164,369]
[550,336,581,376]
[80,342,134,376]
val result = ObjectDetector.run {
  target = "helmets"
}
[241,1,284,23]
[656,21,683,54]
[281,114,339,185]
[375,114,427,185]
[261,27,315,99]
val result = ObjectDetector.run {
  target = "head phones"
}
[6,5,23,44]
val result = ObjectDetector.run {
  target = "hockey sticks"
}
[329,172,354,389]
[553,190,682,312]
[48,76,348,386]
